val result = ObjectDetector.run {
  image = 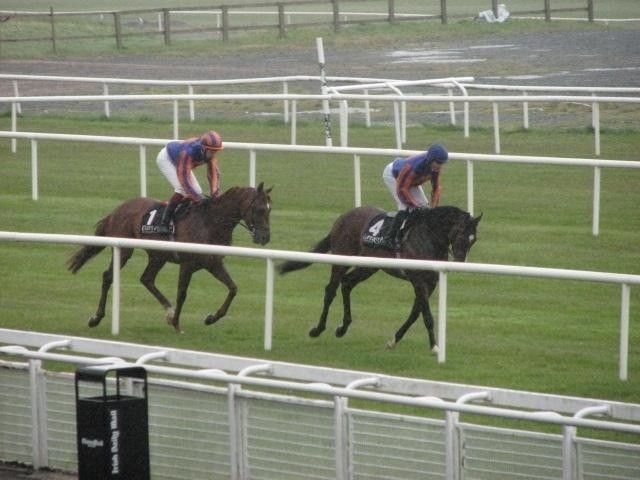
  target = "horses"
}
[65,181,275,329]
[275,204,484,356]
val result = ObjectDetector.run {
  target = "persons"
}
[154,128,222,231]
[381,144,448,253]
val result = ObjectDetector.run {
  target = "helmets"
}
[427,144,448,164]
[199,130,224,151]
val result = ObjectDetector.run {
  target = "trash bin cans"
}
[74,363,150,480]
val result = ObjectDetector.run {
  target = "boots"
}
[381,210,409,251]
[157,193,184,234]
